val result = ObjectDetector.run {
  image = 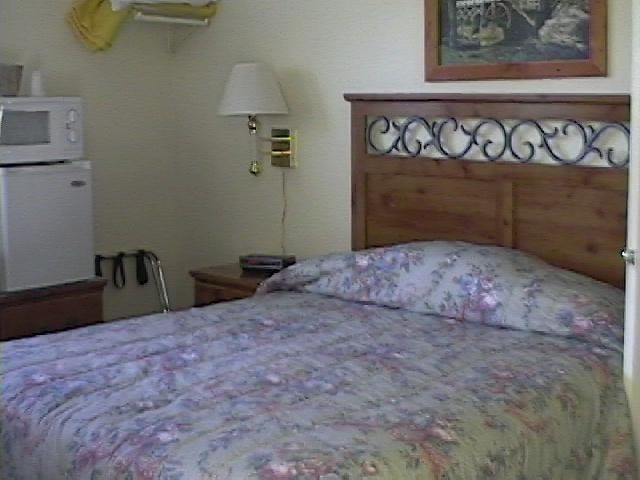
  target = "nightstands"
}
[184,254,286,314]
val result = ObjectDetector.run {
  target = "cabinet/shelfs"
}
[0,272,109,354]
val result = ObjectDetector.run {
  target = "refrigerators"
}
[1,163,98,294]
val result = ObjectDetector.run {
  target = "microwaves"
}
[0,96,84,163]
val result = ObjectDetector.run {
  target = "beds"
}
[0,86,640,480]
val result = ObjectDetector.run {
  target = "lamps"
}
[213,59,300,178]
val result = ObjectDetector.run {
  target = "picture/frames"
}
[421,0,610,85]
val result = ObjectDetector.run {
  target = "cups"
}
[0,64,23,91]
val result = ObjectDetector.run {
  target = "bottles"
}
[29,69,44,94]
